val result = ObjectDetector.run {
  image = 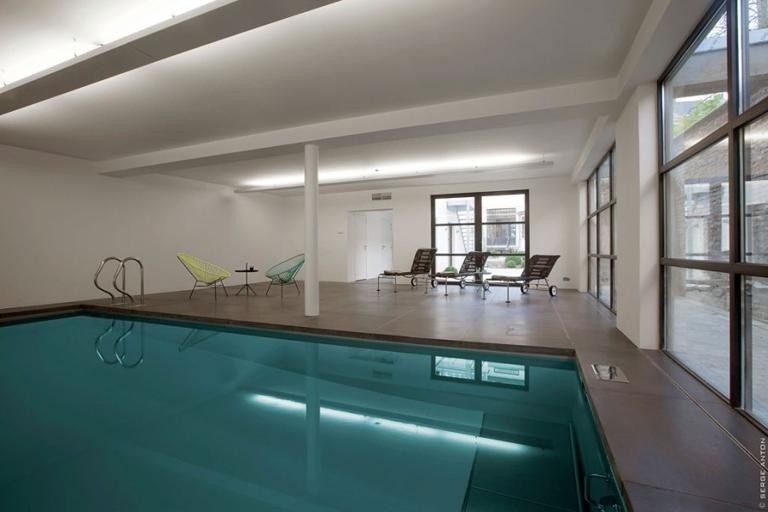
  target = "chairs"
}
[176,252,228,301]
[378,248,561,305]
[265,254,306,299]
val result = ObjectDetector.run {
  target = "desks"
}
[234,268,259,295]
